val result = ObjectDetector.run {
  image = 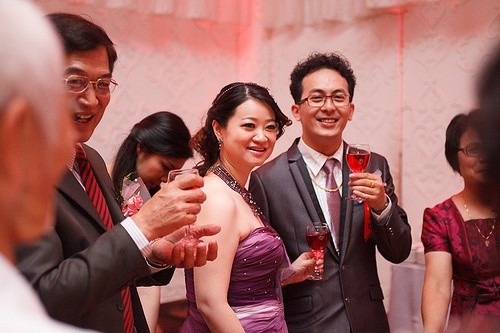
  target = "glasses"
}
[62,74,118,98]
[458,142,482,157]
[298,92,350,107]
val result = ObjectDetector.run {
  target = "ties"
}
[322,159,343,249]
[73,143,135,333]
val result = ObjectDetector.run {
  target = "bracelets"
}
[373,203,390,213]
[147,259,168,268]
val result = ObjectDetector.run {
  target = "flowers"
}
[115,171,144,218]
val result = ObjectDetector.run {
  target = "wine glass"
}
[305,221,332,281]
[344,143,371,200]
[167,169,206,258]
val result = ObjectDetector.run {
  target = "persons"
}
[177,82,326,333]
[111,110,194,333]
[248,50,412,333]
[0,0,94,333]
[420,110,500,333]
[15,13,222,333]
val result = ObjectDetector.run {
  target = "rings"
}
[370,179,374,188]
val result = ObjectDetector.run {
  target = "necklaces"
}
[215,164,258,207]
[311,178,342,191]
[462,192,497,246]
